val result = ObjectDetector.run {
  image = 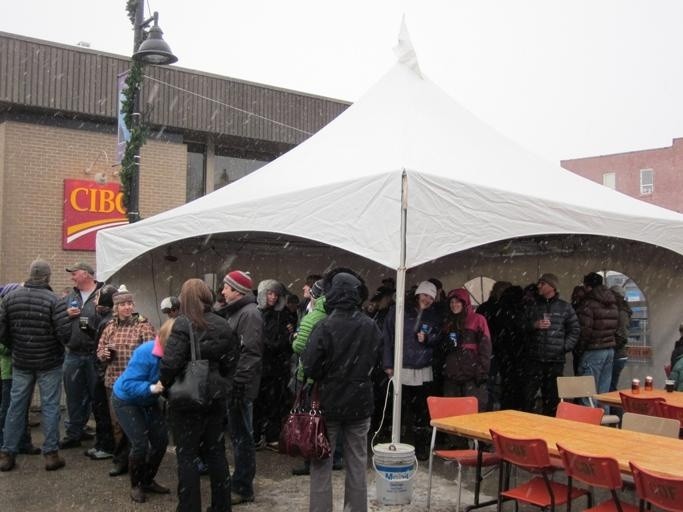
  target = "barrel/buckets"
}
[371,442,419,506]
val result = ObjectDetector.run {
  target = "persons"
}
[52,263,104,455]
[161,277,240,511]
[86,285,116,460]
[1,258,71,471]
[111,318,175,505]
[302,268,383,511]
[161,273,633,473]
[1,344,42,456]
[216,272,264,506]
[664,356,683,392]
[97,286,157,477]
[671,324,683,360]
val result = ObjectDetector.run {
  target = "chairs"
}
[426,363,683,512]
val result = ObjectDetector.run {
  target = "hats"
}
[161,297,181,313]
[30,259,50,276]
[223,270,253,294]
[414,281,438,300]
[111,284,133,304]
[257,279,286,312]
[583,272,603,287]
[309,278,326,299]
[370,286,391,302]
[540,273,558,290]
[66,262,94,274]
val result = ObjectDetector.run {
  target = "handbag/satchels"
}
[278,412,332,461]
[168,359,210,411]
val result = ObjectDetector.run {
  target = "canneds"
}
[449,332,457,347]
[420,324,429,334]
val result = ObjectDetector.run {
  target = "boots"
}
[141,453,169,494]
[415,429,428,460]
[128,454,146,503]
[18,427,41,455]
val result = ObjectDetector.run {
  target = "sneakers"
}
[0,451,17,471]
[85,447,113,460]
[60,437,81,449]
[255,440,279,453]
[108,463,129,476]
[44,450,65,471]
[231,492,254,505]
[292,462,310,475]
[333,451,344,470]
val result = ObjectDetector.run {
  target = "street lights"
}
[127,0,178,224]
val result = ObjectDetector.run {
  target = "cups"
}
[665,379,675,392]
[623,344,649,363]
[448,332,458,348]
[419,323,432,334]
[542,312,552,329]
[644,375,653,390]
[631,378,641,394]
[79,316,90,329]
[70,300,81,318]
